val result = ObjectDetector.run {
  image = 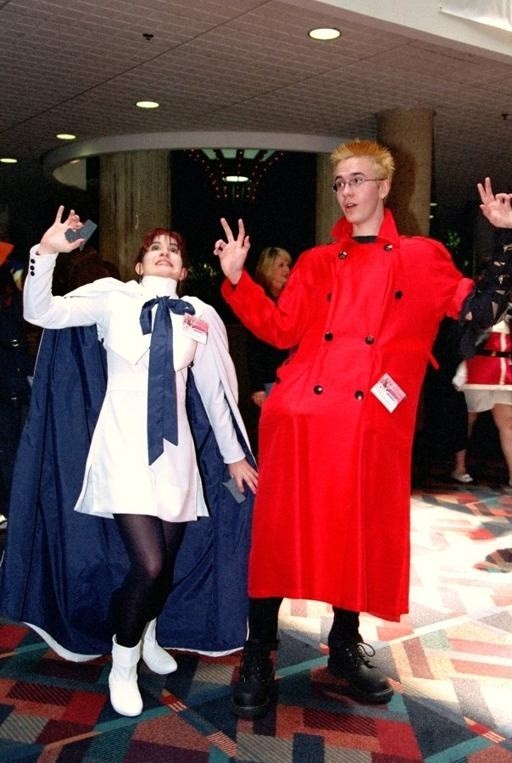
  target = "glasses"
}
[331,177,385,192]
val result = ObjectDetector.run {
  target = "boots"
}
[326,634,393,706]
[143,617,177,674]
[232,634,280,719]
[109,634,143,717]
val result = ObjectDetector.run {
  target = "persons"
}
[248,246,295,408]
[21,204,261,715]
[211,138,512,719]
[449,285,512,484]
